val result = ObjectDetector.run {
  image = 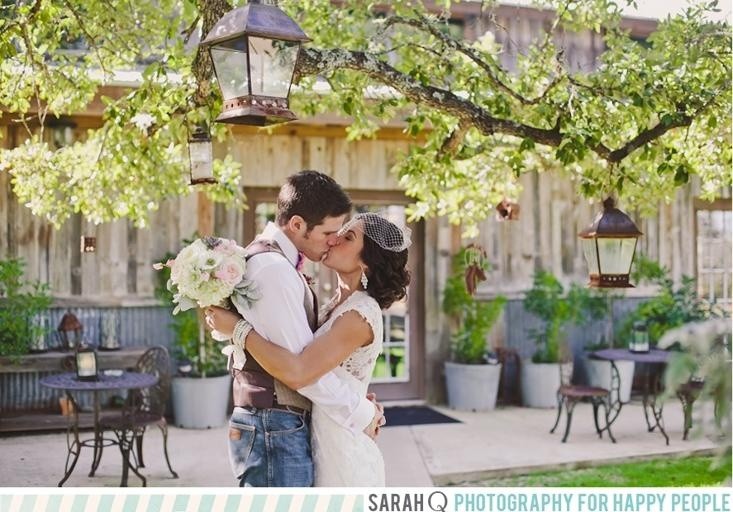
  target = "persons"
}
[203,210,413,488]
[227,169,387,488]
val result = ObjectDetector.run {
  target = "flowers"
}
[152,236,262,315]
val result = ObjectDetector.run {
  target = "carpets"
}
[369,402,463,426]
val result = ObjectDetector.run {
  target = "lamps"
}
[53,309,86,352]
[195,0,313,129]
[180,90,217,190]
[579,162,643,290]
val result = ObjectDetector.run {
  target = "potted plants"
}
[439,246,506,412]
[581,275,639,404]
[153,230,232,429]
[509,274,610,410]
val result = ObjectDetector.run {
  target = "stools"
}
[549,384,616,445]
[669,375,727,440]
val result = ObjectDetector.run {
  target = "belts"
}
[242,398,311,417]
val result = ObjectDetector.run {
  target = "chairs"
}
[113,344,182,478]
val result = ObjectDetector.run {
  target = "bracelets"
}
[221,318,253,371]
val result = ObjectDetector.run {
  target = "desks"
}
[585,348,691,445]
[42,368,157,486]
